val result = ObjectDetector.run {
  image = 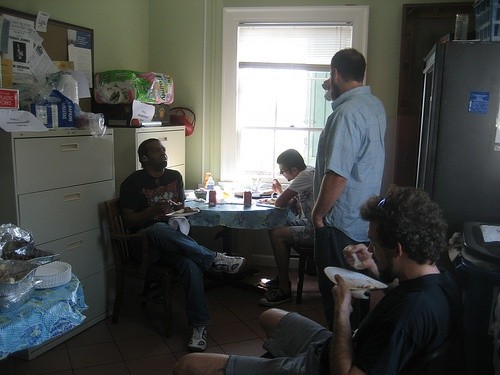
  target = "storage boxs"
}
[90,89,170,128]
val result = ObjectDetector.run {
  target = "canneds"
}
[209,190,216,207]
[243,191,251,207]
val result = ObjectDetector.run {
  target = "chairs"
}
[105,200,191,339]
[293,244,315,305]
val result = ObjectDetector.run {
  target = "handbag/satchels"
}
[169,110,194,136]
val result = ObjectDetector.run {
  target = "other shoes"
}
[256,289,293,305]
[257,274,291,288]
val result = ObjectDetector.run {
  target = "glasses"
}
[280,169,288,174]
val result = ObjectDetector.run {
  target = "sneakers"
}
[187,326,208,351]
[208,252,245,274]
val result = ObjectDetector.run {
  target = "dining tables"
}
[183,190,296,254]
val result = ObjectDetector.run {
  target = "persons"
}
[118,139,247,352]
[257,150,314,307]
[176,184,464,375]
[310,49,388,335]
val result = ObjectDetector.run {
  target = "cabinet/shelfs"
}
[0,128,117,361]
[109,125,186,200]
